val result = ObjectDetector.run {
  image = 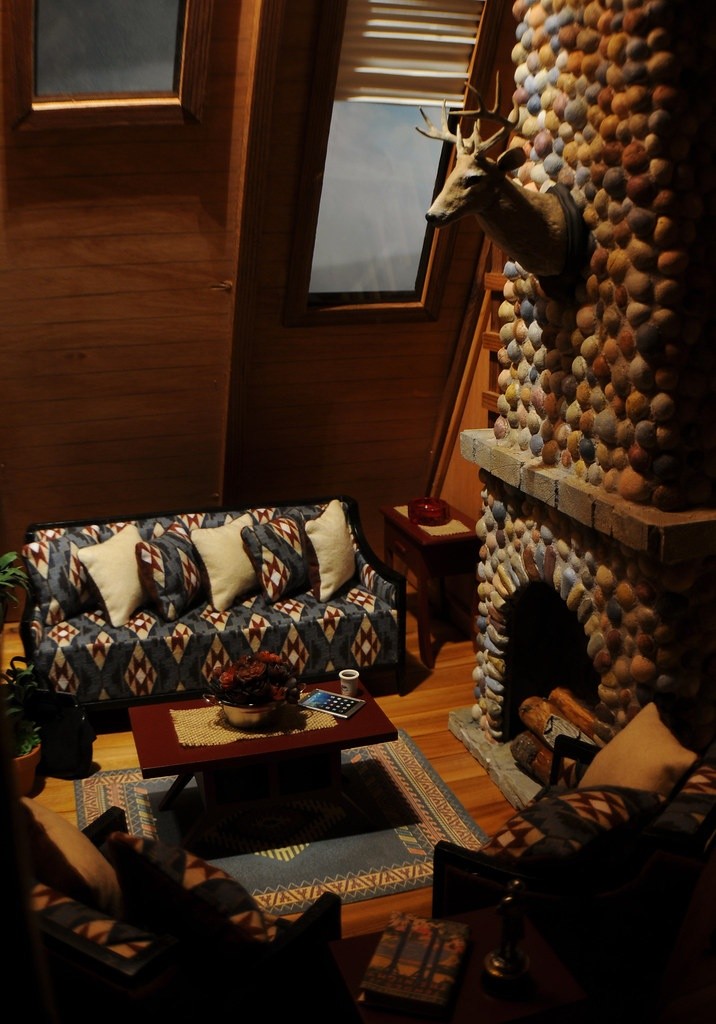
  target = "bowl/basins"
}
[407,498,450,525]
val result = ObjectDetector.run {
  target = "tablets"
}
[298,688,366,718]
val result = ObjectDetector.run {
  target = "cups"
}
[339,669,359,697]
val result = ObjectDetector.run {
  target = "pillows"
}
[483,785,662,872]
[578,700,701,816]
[74,523,148,628]
[241,513,309,608]
[190,512,261,611]
[135,521,207,622]
[101,830,266,954]
[301,499,355,604]
[0,797,119,911]
[22,524,100,627]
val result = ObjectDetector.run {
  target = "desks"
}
[378,498,485,669]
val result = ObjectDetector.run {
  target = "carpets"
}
[74,728,490,916]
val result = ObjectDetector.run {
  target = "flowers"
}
[209,649,299,704]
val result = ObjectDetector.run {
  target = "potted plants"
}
[0,666,42,799]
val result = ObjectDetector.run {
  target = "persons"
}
[492,879,527,959]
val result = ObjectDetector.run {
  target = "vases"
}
[202,683,307,732]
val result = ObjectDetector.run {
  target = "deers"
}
[414,70,569,279]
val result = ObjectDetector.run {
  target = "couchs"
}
[431,735,716,983]
[0,807,343,1024]
[19,495,406,714]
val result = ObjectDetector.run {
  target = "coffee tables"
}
[127,678,398,811]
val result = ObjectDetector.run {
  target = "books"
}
[355,910,470,1017]
